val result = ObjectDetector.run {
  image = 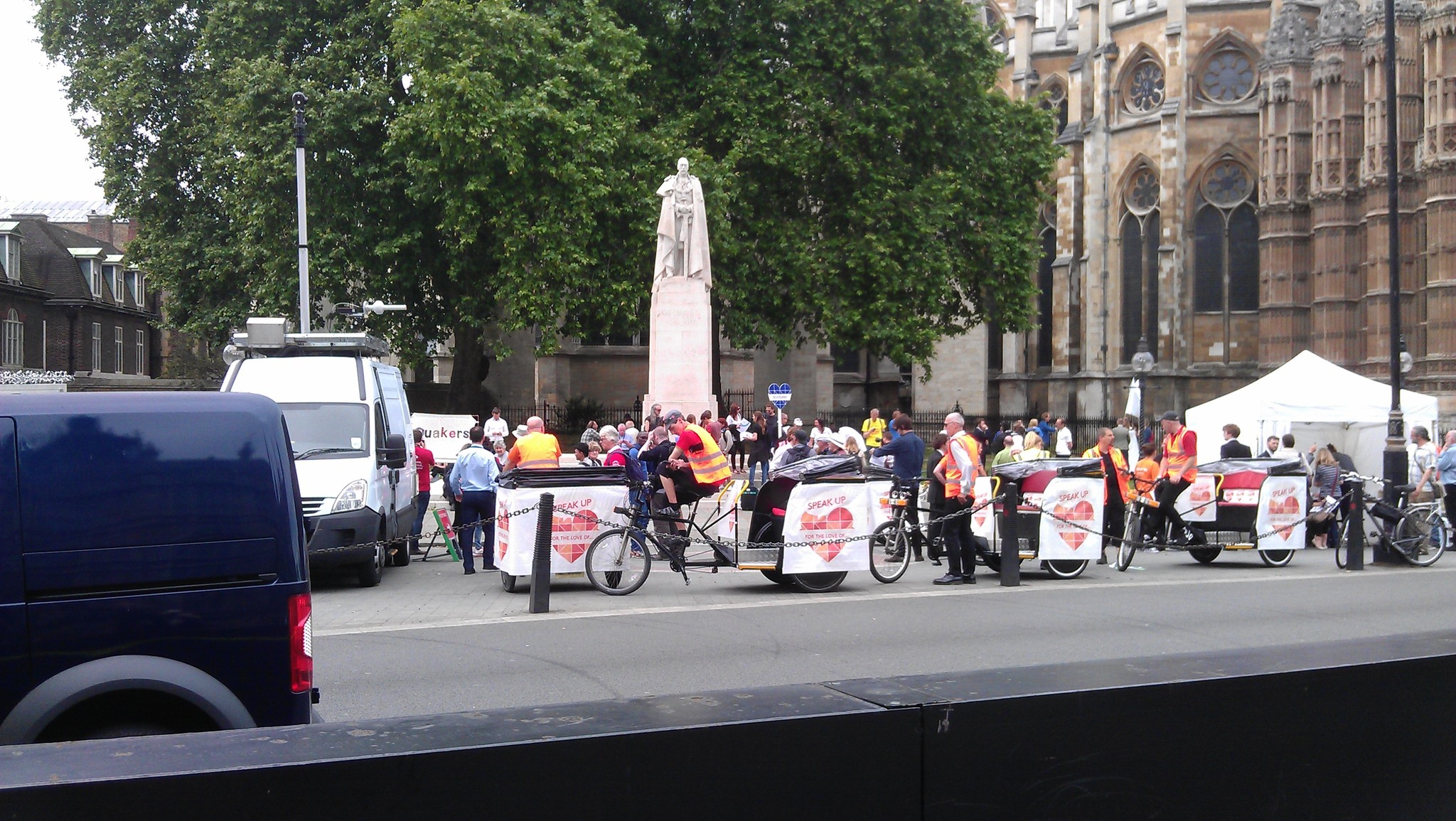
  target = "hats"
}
[1155,411,1179,422]
[574,442,590,452]
[663,409,681,430]
[794,430,846,450]
[512,425,529,439]
[794,418,803,426]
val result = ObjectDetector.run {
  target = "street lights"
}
[1131,333,1157,457]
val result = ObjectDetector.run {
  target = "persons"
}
[653,157,712,292]
[413,402,1455,587]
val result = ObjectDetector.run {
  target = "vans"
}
[0,371,321,748]
[204,317,419,587]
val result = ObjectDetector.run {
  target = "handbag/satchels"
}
[1432,483,1443,498]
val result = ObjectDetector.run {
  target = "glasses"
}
[944,422,953,426]
[737,408,740,410]
[655,409,660,411]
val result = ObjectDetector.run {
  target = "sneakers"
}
[653,507,681,519]
[664,539,691,547]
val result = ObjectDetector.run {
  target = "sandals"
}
[733,469,746,474]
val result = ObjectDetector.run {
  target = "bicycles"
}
[1336,471,1447,568]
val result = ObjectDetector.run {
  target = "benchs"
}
[1212,467,1281,508]
[494,461,628,494]
[991,455,1106,510]
[764,452,871,515]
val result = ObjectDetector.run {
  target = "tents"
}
[1186,350,1438,545]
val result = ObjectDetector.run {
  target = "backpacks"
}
[848,449,862,473]
[662,441,688,463]
[608,451,644,491]
[787,446,810,464]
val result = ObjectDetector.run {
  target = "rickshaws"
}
[495,461,636,594]
[867,456,1108,581]
[584,453,865,596]
[1113,457,1307,572]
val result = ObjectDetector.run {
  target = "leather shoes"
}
[933,573,977,585]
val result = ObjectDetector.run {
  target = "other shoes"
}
[410,546,498,575]
[915,555,923,561]
[1096,526,1196,564]
[1311,540,1327,550]
[884,555,902,562]
[1039,561,1047,570]
[630,550,644,558]
[650,554,669,560]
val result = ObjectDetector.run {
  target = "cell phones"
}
[663,461,679,469]
[420,440,424,446]
